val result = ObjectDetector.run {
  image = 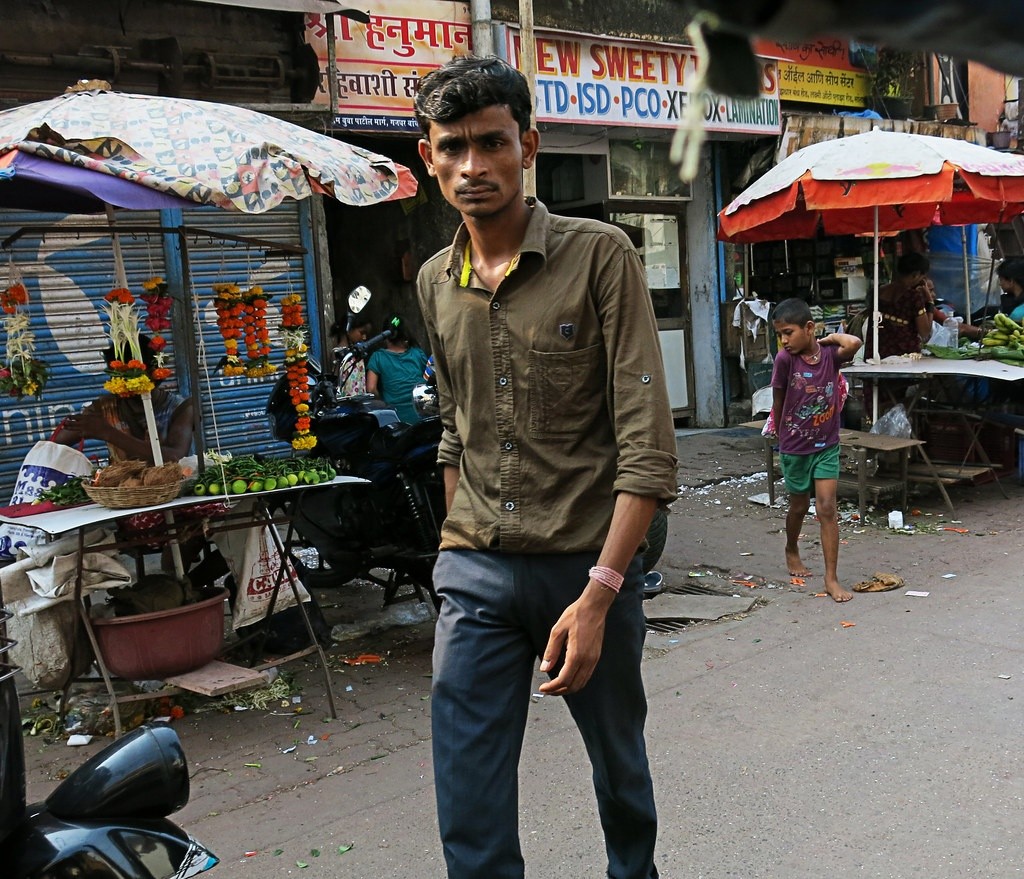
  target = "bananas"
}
[982,312,1024,349]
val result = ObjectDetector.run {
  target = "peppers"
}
[193,456,333,484]
[40,475,94,505]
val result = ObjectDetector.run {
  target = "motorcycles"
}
[262,284,444,614]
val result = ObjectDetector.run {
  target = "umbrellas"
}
[0,79,418,579]
[717,126,1024,428]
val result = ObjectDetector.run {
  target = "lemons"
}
[195,469,336,495]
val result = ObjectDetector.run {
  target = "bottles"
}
[943,311,958,348]
[734,268,741,288]
[728,243,739,262]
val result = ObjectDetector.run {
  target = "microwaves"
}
[816,276,868,303]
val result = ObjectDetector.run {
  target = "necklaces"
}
[806,343,820,362]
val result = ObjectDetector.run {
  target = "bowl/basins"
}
[89,587,231,681]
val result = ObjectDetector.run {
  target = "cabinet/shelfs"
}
[739,420,926,526]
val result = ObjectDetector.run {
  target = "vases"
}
[935,103,959,122]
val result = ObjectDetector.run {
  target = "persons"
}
[48,334,237,594]
[328,313,437,424]
[923,278,991,338]
[412,54,679,879]
[770,298,862,602]
[995,259,1024,327]
[846,251,935,404]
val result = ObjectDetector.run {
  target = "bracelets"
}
[925,301,934,314]
[977,327,996,340]
[589,566,624,593]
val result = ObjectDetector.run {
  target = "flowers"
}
[0,284,317,450]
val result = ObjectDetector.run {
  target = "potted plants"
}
[870,46,924,119]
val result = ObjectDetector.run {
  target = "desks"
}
[838,354,1024,501]
[0,468,371,740]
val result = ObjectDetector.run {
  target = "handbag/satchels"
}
[209,498,311,630]
[0,415,94,558]
[762,372,852,441]
[926,317,963,348]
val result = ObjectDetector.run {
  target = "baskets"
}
[81,481,182,507]
